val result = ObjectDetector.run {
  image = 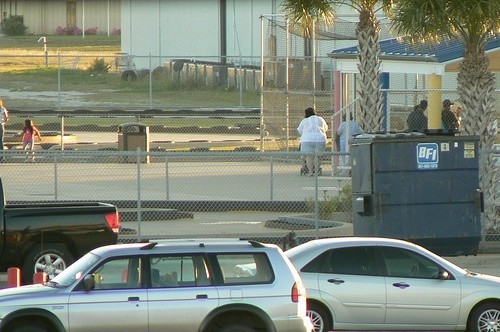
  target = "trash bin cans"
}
[118,123,147,163]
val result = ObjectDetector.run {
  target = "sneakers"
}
[24,158,37,163]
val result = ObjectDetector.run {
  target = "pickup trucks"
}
[0,176,119,288]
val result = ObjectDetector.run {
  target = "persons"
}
[406,99,461,130]
[0,99,9,163]
[297,107,328,177]
[337,112,364,166]
[20,119,41,163]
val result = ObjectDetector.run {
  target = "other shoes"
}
[308,172,314,177]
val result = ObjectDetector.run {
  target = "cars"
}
[235,236,500,332]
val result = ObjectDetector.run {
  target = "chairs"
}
[129,268,160,288]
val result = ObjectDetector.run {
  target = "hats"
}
[443,99,454,105]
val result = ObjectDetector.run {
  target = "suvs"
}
[0,239,314,332]
